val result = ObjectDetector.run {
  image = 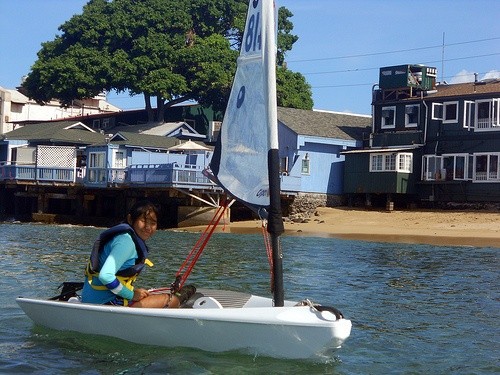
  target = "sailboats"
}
[15,0,351,360]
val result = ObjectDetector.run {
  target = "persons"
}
[81,202,196,307]
